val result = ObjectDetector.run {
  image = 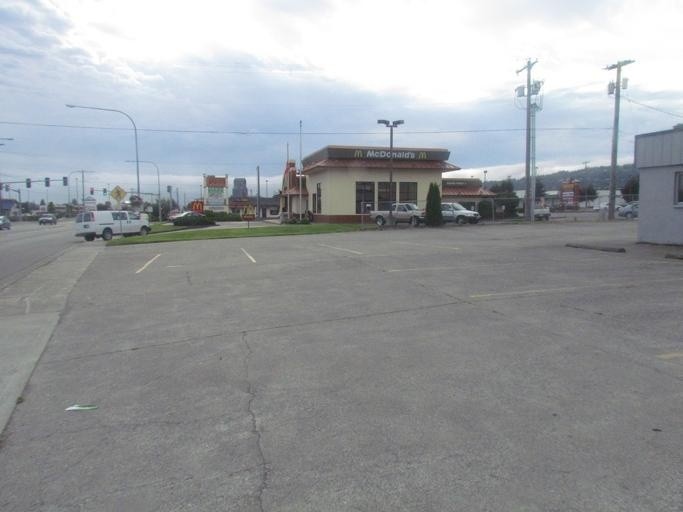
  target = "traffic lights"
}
[102,188,107,196]
[89,188,93,195]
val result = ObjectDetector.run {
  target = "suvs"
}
[440,202,481,226]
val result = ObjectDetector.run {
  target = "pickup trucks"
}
[368,200,425,228]
[533,204,550,220]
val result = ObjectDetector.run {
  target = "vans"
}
[72,210,151,240]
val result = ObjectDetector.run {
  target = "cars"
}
[167,210,205,223]
[0,215,11,230]
[37,214,57,226]
[592,199,639,218]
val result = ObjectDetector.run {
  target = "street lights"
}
[123,159,161,221]
[482,169,487,188]
[376,119,405,227]
[0,137,15,141]
[63,104,140,198]
